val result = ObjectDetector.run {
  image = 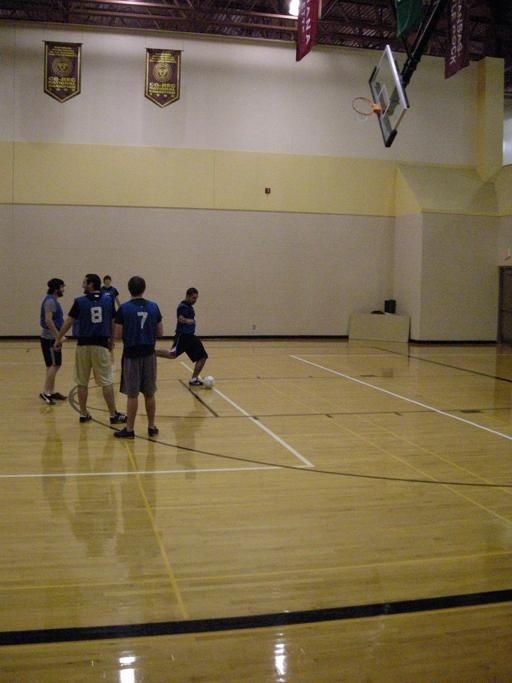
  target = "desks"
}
[349,312,409,343]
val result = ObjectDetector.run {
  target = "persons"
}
[112,276,163,439]
[154,288,208,386]
[38,277,67,405]
[101,275,122,351]
[53,274,127,424]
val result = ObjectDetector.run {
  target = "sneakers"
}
[114,426,135,439]
[148,426,159,436]
[189,376,203,385]
[47,392,66,399]
[39,392,56,404]
[80,414,92,422]
[110,410,127,424]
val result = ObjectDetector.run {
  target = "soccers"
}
[203,375,215,389]
